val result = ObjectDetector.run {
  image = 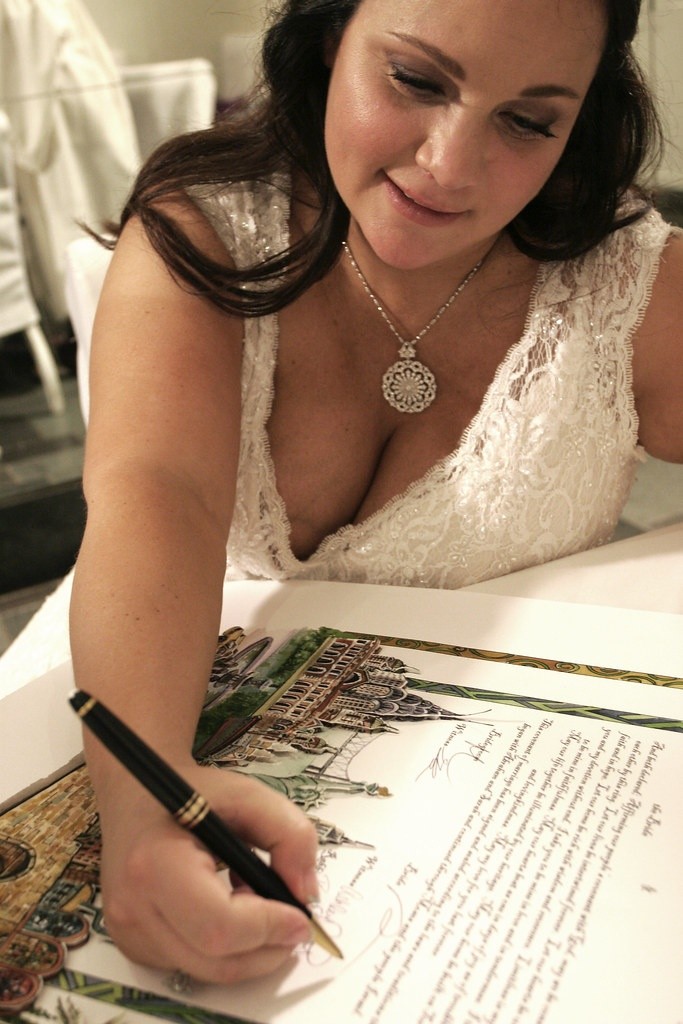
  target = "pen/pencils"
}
[67,689,347,966]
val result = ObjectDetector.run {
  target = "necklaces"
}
[342,241,486,414]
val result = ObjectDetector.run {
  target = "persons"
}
[67,0,683,980]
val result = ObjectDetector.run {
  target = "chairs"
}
[0,110,64,417]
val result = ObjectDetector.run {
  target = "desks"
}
[454,511,683,606]
